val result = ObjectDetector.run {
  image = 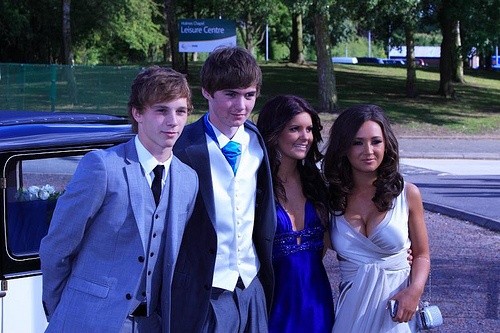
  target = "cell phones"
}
[387,300,398,318]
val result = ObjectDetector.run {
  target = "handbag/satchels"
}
[415,300,444,330]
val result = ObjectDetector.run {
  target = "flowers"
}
[18,184,59,200]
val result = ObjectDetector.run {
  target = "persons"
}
[322,104,431,333]
[39,65,200,333]
[173,48,277,333]
[257,96,413,333]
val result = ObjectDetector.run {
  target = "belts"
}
[133,301,147,317]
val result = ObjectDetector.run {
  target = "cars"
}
[332,54,430,71]
[0,109,136,333]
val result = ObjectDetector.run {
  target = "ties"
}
[204,111,240,173]
[151,165,164,204]
